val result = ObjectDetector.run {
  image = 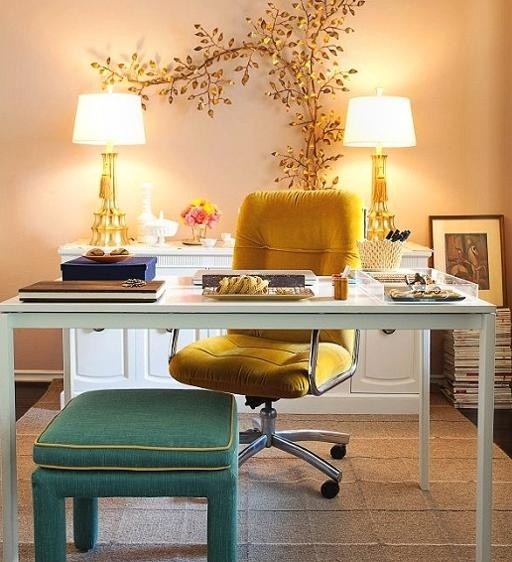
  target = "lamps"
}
[342,95,416,240]
[71,92,147,246]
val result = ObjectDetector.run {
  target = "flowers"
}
[179,197,222,230]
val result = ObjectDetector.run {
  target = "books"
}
[440,307,512,410]
[18,281,166,303]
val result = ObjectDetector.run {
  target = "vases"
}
[192,226,207,241]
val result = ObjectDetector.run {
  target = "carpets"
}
[15,376,512,562]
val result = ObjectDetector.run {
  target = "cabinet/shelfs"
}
[331,256,431,417]
[198,256,350,415]
[59,255,208,412]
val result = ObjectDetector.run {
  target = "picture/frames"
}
[429,214,508,309]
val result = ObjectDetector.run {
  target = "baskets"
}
[356,239,406,272]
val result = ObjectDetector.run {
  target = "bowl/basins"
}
[200,238,216,248]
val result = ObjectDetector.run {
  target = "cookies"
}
[87,247,105,257]
[110,248,130,257]
[216,276,269,295]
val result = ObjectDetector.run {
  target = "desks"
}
[0,274,496,562]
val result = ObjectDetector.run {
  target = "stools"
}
[31,388,240,561]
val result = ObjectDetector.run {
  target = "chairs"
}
[170,190,364,499]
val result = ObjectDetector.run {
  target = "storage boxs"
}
[60,257,157,282]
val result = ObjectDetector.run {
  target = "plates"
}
[82,253,135,263]
[201,286,315,301]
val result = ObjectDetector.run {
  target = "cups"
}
[334,277,348,300]
[220,232,231,242]
